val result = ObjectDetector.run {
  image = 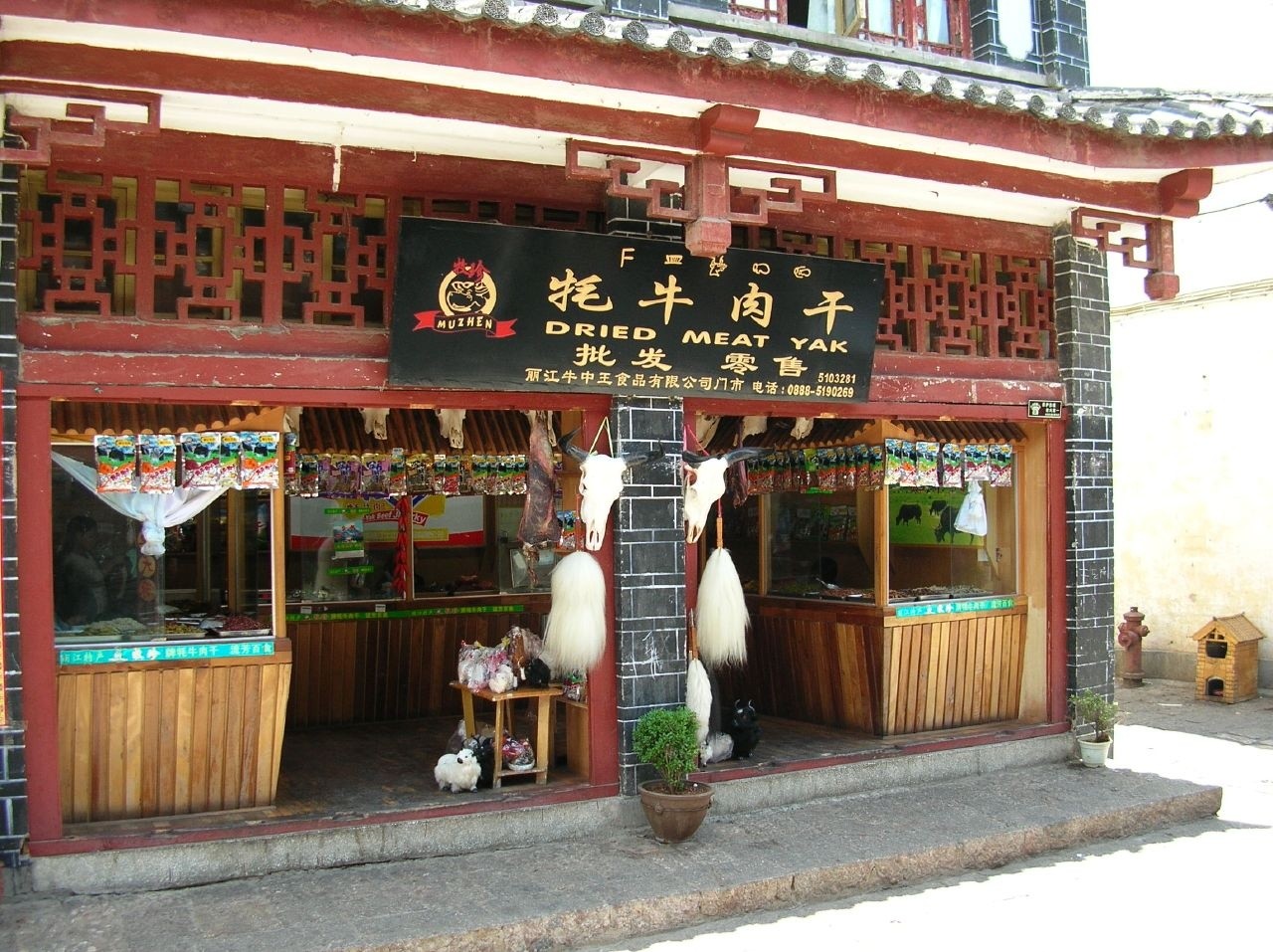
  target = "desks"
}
[450,678,564,788]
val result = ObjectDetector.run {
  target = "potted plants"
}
[631,705,716,846]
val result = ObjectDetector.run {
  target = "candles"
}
[1067,688,1120,767]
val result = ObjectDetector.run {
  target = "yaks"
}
[561,424,761,552]
[896,500,974,545]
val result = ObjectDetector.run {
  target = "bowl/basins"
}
[507,760,535,770]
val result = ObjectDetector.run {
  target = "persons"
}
[811,557,838,586]
[55,515,118,625]
